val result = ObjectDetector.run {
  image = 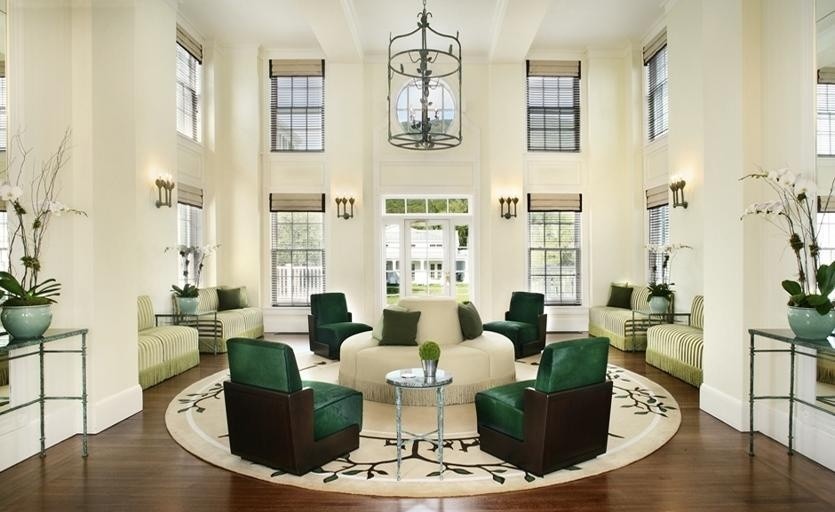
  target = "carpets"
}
[160,352,682,498]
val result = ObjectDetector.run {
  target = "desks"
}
[0,324,95,466]
[154,311,220,359]
[745,323,834,461]
[632,309,691,353]
[384,366,454,481]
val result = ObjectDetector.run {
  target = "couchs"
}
[472,331,617,478]
[138,293,202,394]
[482,287,551,360]
[172,283,267,356]
[223,332,365,479]
[334,296,519,411]
[587,279,678,352]
[643,291,705,391]
[307,290,374,360]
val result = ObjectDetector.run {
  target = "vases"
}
[646,297,675,314]
[169,298,201,314]
[1,308,61,342]
[783,307,834,345]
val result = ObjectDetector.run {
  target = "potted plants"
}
[418,340,442,379]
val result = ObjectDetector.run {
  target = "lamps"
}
[496,188,521,219]
[147,167,176,210]
[333,188,360,223]
[666,165,696,211]
[384,1,465,153]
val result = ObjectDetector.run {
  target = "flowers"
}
[643,234,695,298]
[739,162,834,307]
[159,240,222,296]
[0,122,90,307]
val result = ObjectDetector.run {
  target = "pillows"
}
[457,300,483,340]
[377,306,426,346]
[215,287,244,313]
[606,285,635,310]
